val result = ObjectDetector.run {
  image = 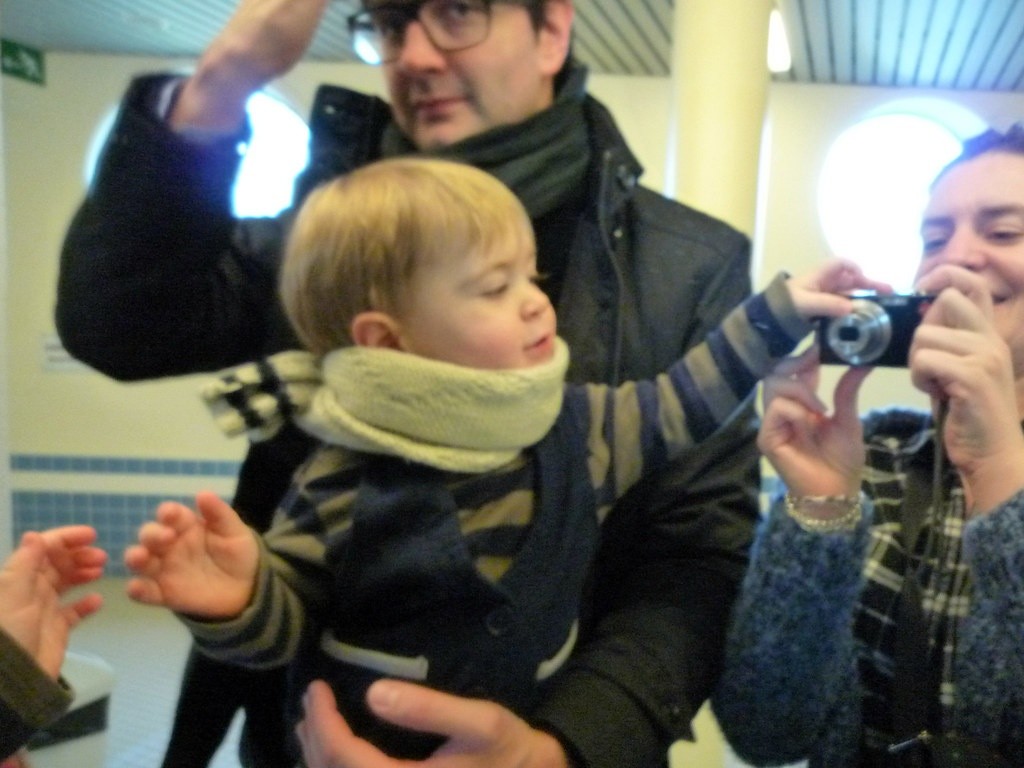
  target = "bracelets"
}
[783,491,865,533]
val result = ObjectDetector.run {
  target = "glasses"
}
[349,0,494,66]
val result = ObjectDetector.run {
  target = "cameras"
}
[820,289,937,369]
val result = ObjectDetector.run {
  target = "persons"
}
[0,526,107,767]
[126,159,894,767]
[708,122,1024,768]
[54,0,761,767]
